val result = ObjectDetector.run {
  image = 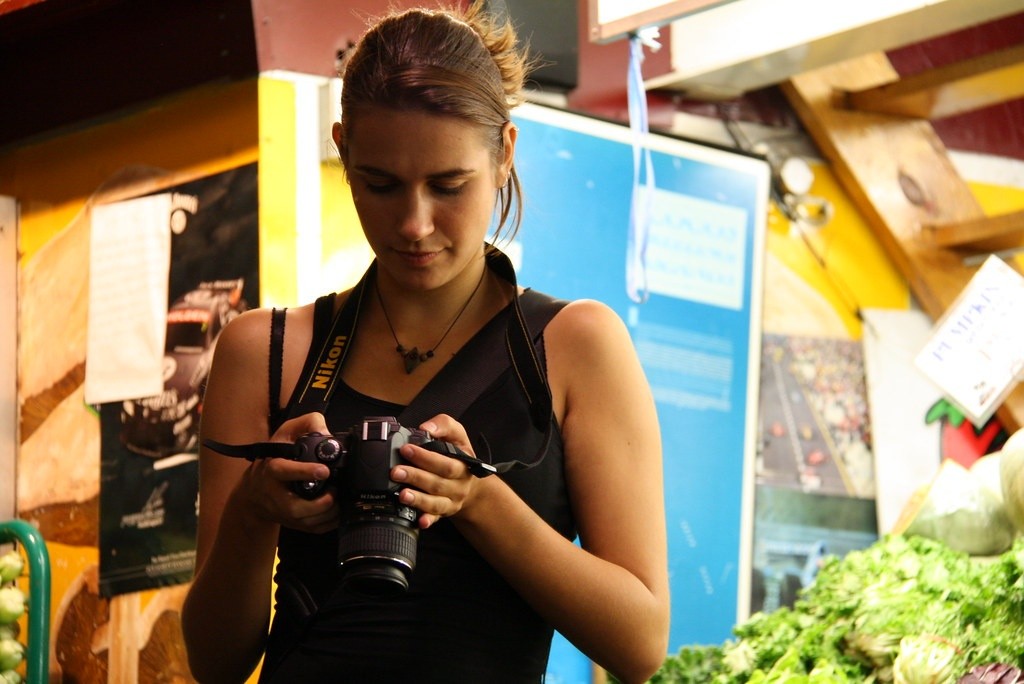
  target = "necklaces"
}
[372,256,487,375]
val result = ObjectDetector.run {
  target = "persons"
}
[182,0,671,684]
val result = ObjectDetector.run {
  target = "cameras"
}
[283,414,435,611]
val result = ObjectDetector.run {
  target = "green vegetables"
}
[601,533,1024,684]
